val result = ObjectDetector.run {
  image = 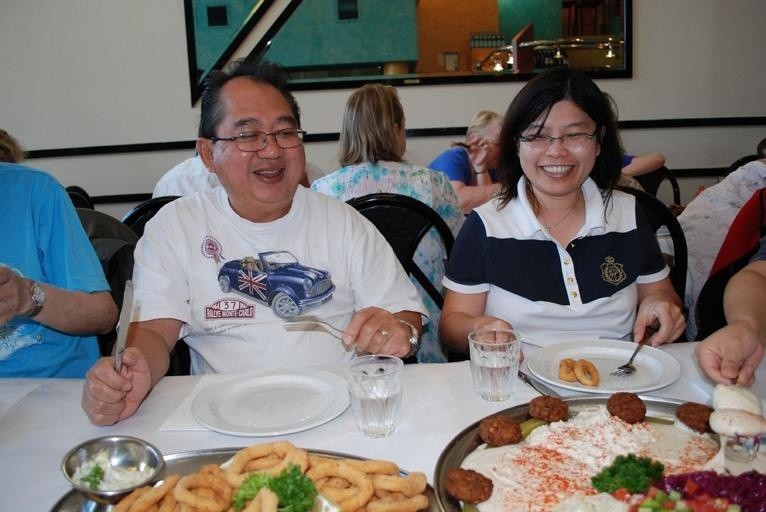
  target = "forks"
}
[609,324,657,377]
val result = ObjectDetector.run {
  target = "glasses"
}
[517,127,599,147]
[210,128,308,152]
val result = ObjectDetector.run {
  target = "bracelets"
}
[474,168,489,174]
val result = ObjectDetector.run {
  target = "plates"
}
[435,392,766,511]
[527,340,682,395]
[47,447,438,511]
[191,367,352,438]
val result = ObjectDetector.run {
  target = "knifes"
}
[115,279,134,370]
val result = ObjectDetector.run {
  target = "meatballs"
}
[528,395,569,423]
[675,401,714,434]
[443,465,494,505]
[478,415,523,447]
[606,391,648,424]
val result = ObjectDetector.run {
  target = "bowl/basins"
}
[60,435,165,499]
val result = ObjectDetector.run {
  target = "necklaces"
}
[531,192,585,235]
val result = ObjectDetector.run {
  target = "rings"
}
[377,327,389,336]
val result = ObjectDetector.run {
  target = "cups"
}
[346,354,404,439]
[468,329,521,404]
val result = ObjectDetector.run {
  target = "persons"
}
[694,189,766,389]
[439,68,686,363]
[311,85,463,364]
[154,155,325,197]
[81,58,432,426]
[622,152,666,176]
[0,130,119,379]
[426,111,506,217]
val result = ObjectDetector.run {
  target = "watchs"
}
[28,279,45,317]
[397,317,420,359]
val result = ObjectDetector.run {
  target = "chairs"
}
[624,164,682,209]
[615,186,688,343]
[347,194,456,311]
[117,196,182,235]
